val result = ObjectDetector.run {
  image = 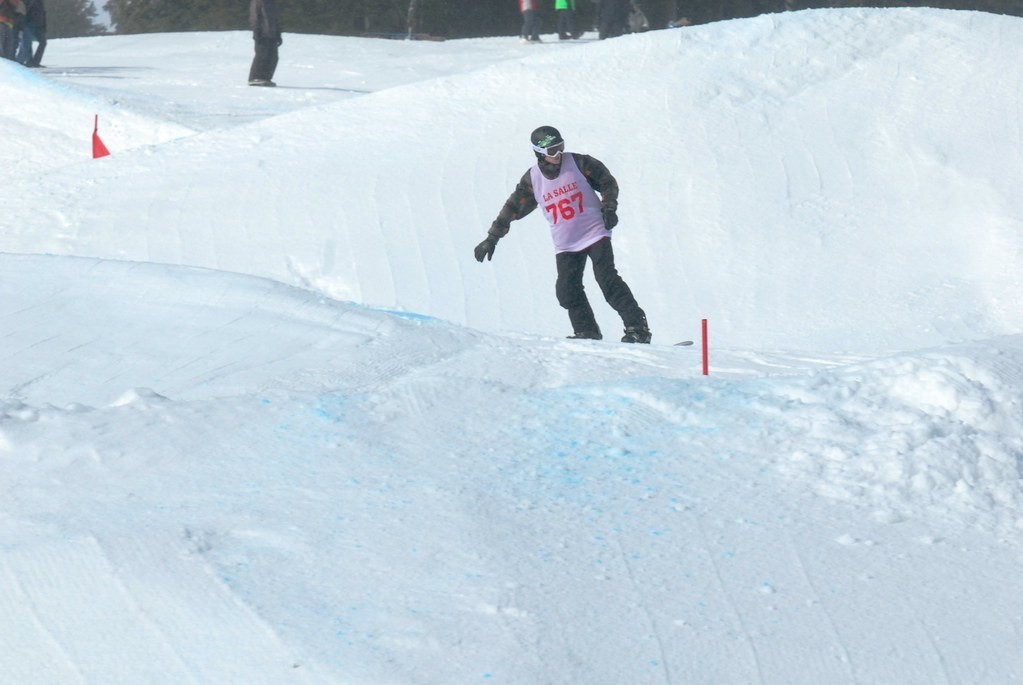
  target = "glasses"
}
[545,138,564,157]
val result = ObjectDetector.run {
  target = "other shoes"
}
[248,78,276,86]
[671,17,692,26]
[529,37,542,43]
[32,63,45,68]
[560,35,572,39]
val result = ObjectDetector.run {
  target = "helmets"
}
[531,126,562,159]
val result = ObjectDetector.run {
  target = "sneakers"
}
[566,331,602,340]
[620,326,652,343]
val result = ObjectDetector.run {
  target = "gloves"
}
[276,31,282,47]
[601,205,618,230]
[253,28,262,44]
[474,233,500,262]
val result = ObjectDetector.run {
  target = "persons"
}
[249,1,283,87]
[592,1,650,39]
[554,0,584,40]
[518,1,543,42]
[0,0,47,67]
[669,1,697,28]
[473,127,652,345]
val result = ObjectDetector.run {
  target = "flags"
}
[92,131,110,160]
